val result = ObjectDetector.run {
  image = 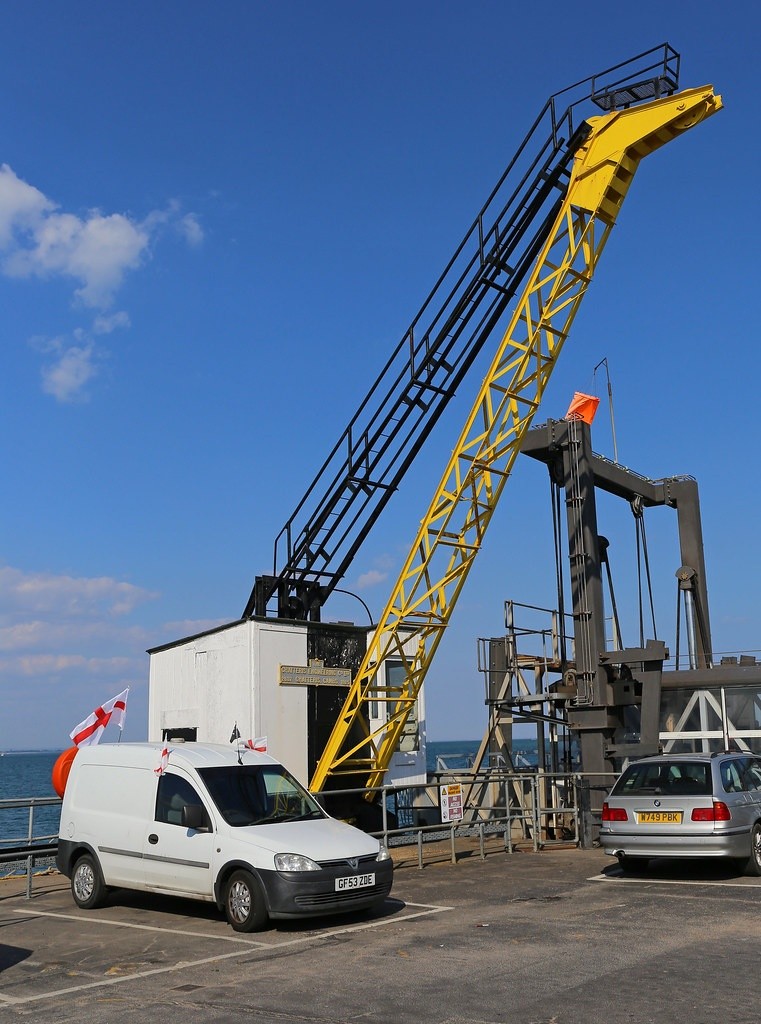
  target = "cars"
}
[597,748,761,878]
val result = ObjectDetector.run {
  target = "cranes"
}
[144,44,727,831]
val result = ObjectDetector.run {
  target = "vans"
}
[51,738,395,931]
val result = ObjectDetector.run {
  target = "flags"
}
[69,688,129,751]
[240,736,267,754]
[154,732,168,776]
[230,724,241,743]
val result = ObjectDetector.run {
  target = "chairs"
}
[166,792,195,823]
